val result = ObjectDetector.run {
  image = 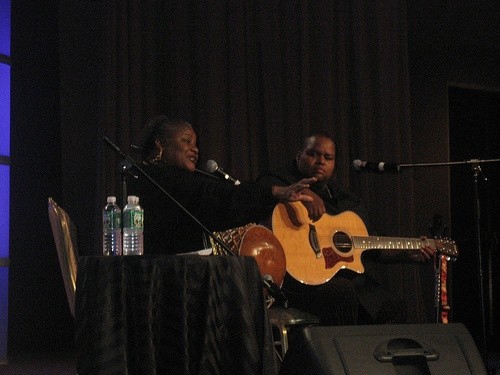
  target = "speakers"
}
[281,323,485,375]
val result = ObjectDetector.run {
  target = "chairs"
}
[48,196,77,316]
[269,305,320,363]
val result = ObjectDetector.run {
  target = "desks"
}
[73,253,277,375]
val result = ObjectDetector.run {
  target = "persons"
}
[121,116,317,254]
[255,131,434,324]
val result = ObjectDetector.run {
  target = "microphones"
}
[352,160,396,172]
[205,160,242,186]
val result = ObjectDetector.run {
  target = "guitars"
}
[271,198,461,286]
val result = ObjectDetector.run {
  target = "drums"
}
[211,221,287,292]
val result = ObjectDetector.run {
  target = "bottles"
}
[102,196,122,256]
[122,195,145,256]
